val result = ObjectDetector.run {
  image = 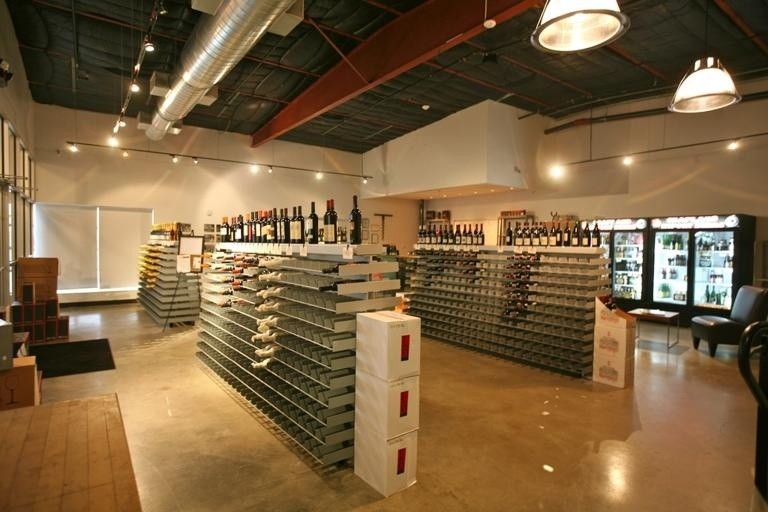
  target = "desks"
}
[1,394,144,512]
[627,307,682,350]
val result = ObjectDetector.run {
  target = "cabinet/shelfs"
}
[126,240,203,329]
[399,244,609,382]
[425,218,451,234]
[191,240,401,477]
[383,255,406,293]
[497,213,535,245]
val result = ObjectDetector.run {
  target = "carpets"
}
[11,336,116,379]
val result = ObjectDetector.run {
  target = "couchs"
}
[688,283,766,359]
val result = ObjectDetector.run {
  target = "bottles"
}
[417,221,484,245]
[615,232,639,299]
[249,256,293,370]
[499,249,539,318]
[137,244,164,289]
[406,248,479,287]
[505,221,601,247]
[323,199,339,243]
[602,233,610,244]
[219,201,319,244]
[348,195,364,245]
[699,255,711,267]
[318,278,365,292]
[200,246,258,308]
[702,274,727,305]
[723,255,733,267]
[697,236,728,250]
[321,262,365,274]
[659,233,687,301]
[149,222,180,241]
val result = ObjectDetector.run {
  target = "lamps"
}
[530,0,630,56]
[549,95,768,179]
[110,1,166,130]
[62,140,373,184]
[663,0,741,114]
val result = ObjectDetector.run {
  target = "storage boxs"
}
[7,258,70,340]
[354,371,420,441]
[0,355,43,410]
[593,324,634,358]
[592,351,634,389]
[353,308,422,382]
[353,424,419,496]
[0,318,14,372]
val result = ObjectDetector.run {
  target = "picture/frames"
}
[361,218,380,245]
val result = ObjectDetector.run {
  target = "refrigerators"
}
[648,214,757,329]
[582,218,648,314]
